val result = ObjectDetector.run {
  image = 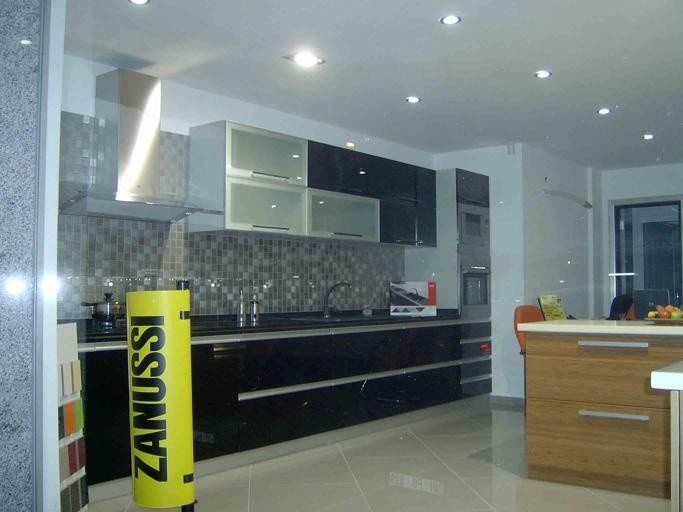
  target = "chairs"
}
[513,305,546,418]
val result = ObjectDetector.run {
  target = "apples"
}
[647,304,683,319]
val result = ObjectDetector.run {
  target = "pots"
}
[80,293,126,321]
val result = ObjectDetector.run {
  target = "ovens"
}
[460,263,490,317]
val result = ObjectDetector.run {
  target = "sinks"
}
[300,316,362,322]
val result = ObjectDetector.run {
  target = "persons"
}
[606,293,638,319]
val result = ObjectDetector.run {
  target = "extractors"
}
[57,68,200,225]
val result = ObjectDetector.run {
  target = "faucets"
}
[323,281,353,319]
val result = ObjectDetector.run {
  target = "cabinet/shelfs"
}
[238,321,462,451]
[516,319,683,500]
[188,119,437,248]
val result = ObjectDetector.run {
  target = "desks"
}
[651,361,683,512]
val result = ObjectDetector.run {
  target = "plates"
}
[644,317,683,326]
